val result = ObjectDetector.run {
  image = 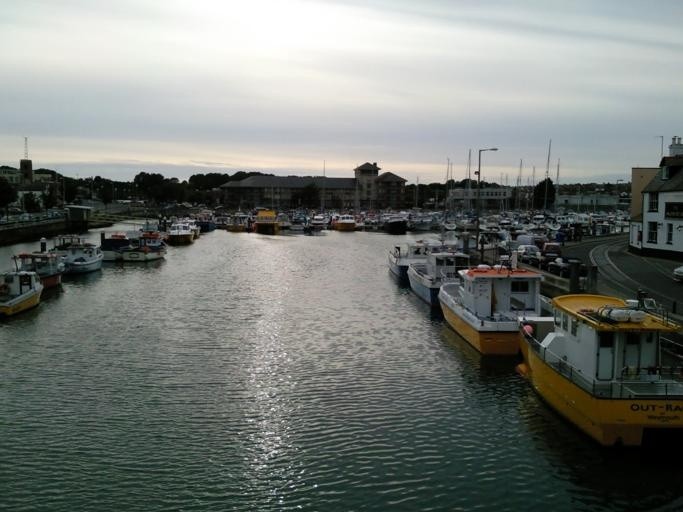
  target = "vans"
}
[517,244,538,256]
[541,242,561,259]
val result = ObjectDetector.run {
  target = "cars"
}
[0,201,75,223]
[671,265,682,282]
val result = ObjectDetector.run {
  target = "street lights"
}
[614,178,623,196]
[473,146,500,244]
[653,134,663,160]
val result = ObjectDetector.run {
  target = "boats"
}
[512,289,683,452]
[14,236,63,294]
[91,228,169,261]
[388,239,450,285]
[434,258,558,360]
[167,138,633,245]
[403,240,478,309]
[47,232,105,272]
[0,269,44,315]
